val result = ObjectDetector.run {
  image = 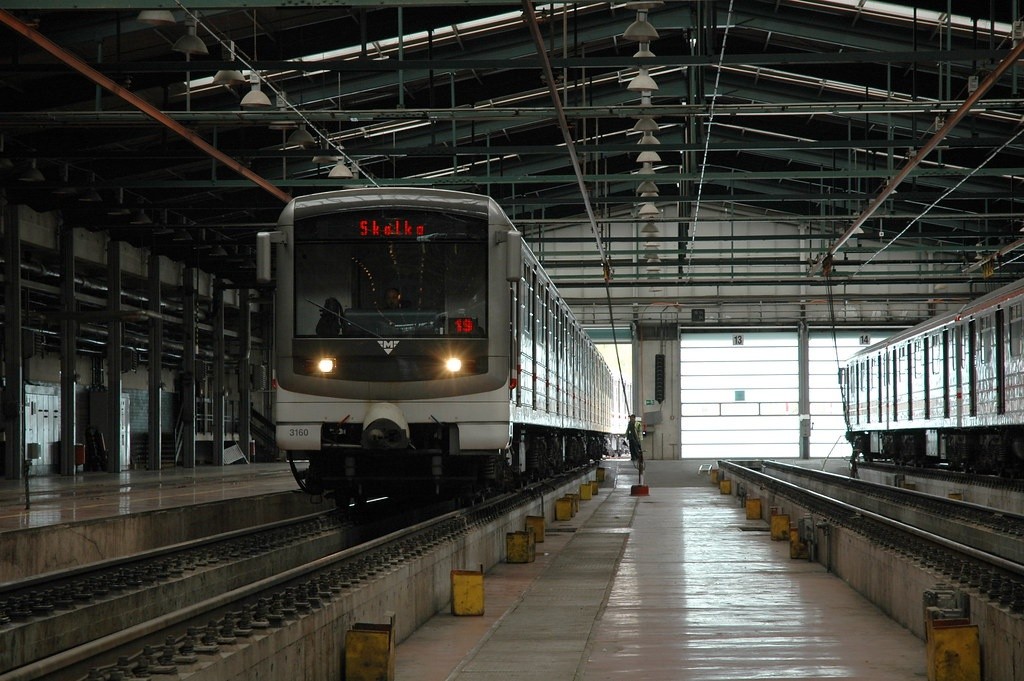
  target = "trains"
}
[839,279,1024,475]
[254,188,633,513]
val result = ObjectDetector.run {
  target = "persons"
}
[622,414,643,460]
[385,288,412,309]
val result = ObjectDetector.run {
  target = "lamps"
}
[135,10,354,178]
[626,2,665,293]
